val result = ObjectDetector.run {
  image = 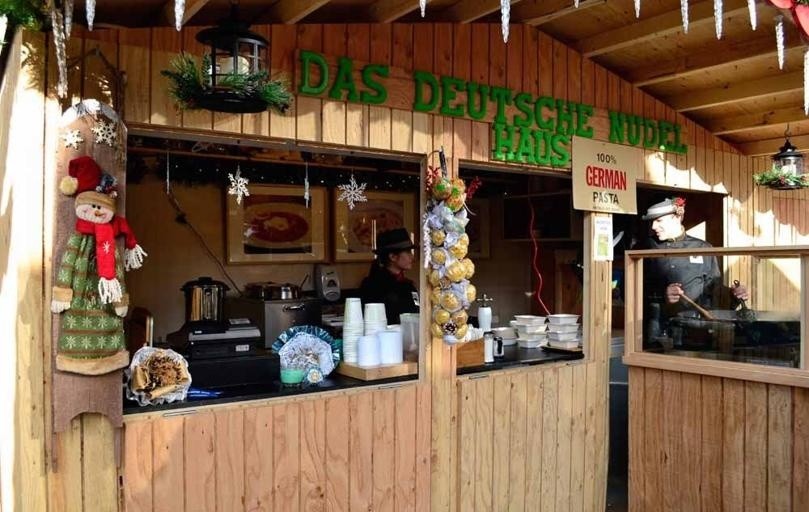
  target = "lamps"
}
[770,133,805,190]
[196,0,271,113]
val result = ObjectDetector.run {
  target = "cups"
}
[340,297,404,369]
[398,311,419,354]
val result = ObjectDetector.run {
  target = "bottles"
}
[493,336,505,357]
[475,294,494,332]
[484,331,495,363]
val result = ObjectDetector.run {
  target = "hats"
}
[377,227,417,254]
[640,196,686,222]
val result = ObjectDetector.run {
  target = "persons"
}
[358,226,420,325]
[617,195,749,329]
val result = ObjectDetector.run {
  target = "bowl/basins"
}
[493,313,582,350]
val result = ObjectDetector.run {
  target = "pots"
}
[663,309,801,353]
[240,281,305,301]
[178,276,231,324]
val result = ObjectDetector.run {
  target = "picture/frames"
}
[465,198,491,259]
[225,184,418,265]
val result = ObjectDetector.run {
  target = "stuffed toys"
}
[49,156,146,376]
